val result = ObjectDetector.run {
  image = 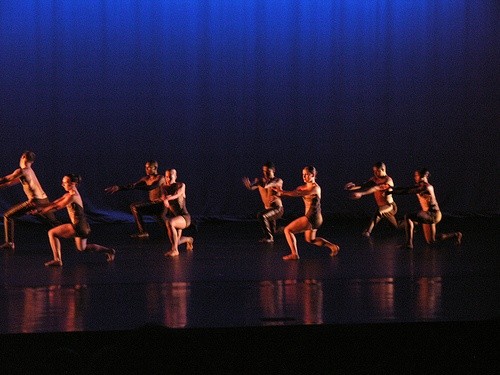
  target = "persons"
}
[105,161,170,238]
[242,162,290,245]
[149,168,194,257]
[344,162,418,237]
[379,167,463,249]
[271,166,340,261]
[0,150,67,249]
[26,172,116,266]
